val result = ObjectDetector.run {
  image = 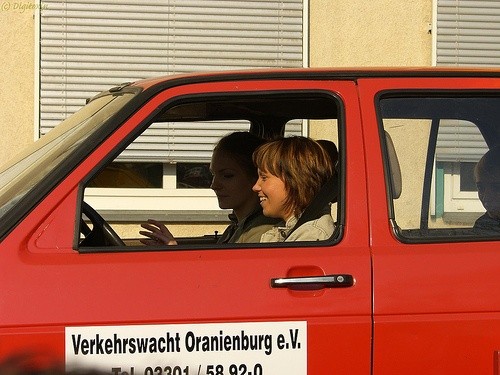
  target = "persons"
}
[209,130,285,244]
[139,133,335,245]
[472,145,500,231]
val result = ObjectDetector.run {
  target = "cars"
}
[0,65,499,375]
